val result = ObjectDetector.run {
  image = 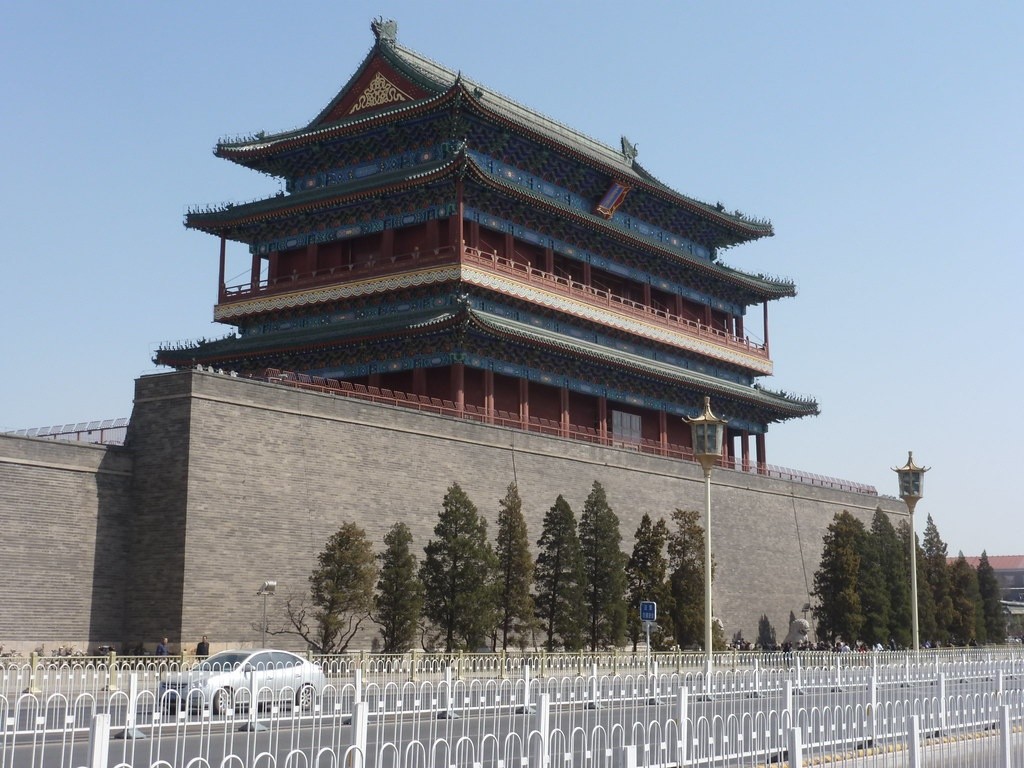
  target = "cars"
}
[159,648,326,714]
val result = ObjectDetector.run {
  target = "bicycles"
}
[0,645,150,667]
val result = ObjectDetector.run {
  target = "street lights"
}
[802,603,811,620]
[256,582,278,649]
[890,450,932,680]
[681,397,734,701]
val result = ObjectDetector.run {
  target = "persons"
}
[155,635,177,672]
[195,635,209,661]
[726,632,1024,666]
